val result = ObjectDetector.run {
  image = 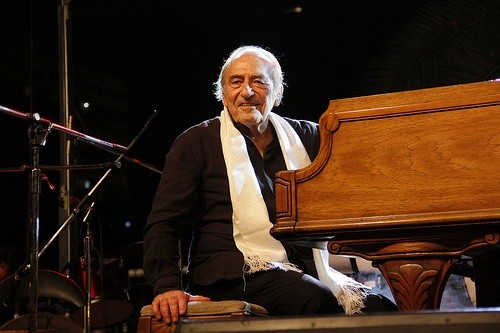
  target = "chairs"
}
[0,248,154,333]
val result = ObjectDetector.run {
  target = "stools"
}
[137,300,269,333]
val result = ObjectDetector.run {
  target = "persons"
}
[139,44,397,315]
